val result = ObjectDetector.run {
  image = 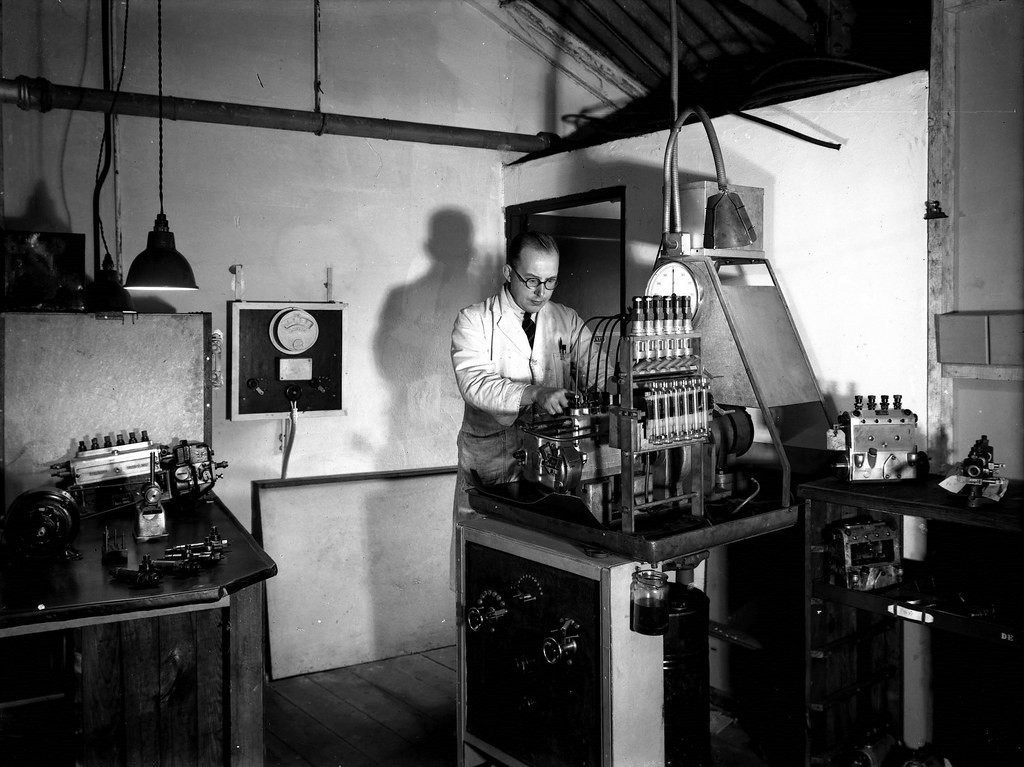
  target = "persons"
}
[449,230,614,593]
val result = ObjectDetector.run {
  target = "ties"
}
[522,309,536,348]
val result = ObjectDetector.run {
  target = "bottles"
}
[91,437,100,450]
[129,432,137,444]
[141,431,150,441]
[104,435,112,448]
[117,434,125,447]
[78,441,87,451]
[632,570,669,636]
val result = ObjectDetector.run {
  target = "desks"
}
[795,469,1024,767]
[0,489,278,767]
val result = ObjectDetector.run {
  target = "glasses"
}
[515,267,560,290]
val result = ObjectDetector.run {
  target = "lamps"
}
[123,0,198,291]
